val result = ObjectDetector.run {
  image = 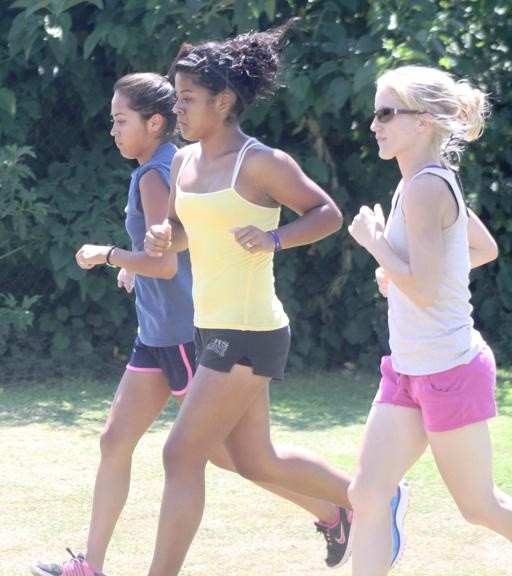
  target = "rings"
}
[247,243,253,248]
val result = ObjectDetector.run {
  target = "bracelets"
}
[268,231,281,253]
[106,245,118,268]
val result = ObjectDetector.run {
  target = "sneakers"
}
[31,546,108,576]
[383,476,410,570]
[313,504,357,569]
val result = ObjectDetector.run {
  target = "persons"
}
[31,41,354,576]
[143,16,410,576]
[347,63,512,576]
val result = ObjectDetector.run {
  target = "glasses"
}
[370,106,434,123]
